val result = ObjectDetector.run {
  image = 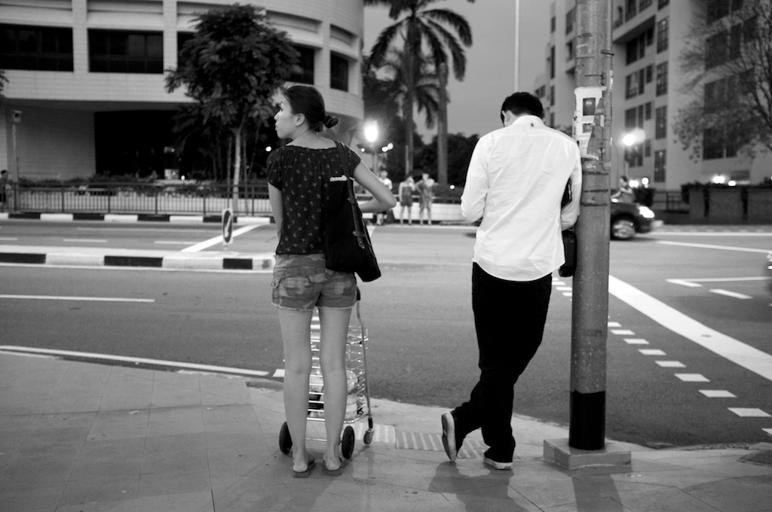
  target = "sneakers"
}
[442,410,466,463]
[484,448,513,469]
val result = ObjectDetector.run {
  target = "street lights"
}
[10,108,24,209]
[621,128,644,203]
[362,117,381,224]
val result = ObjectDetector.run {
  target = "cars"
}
[473,197,655,242]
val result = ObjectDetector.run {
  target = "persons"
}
[0,169,11,212]
[616,174,634,202]
[263,84,397,476]
[440,90,583,471]
[373,168,437,225]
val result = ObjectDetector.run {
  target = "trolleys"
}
[279,271,377,460]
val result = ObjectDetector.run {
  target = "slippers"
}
[292,454,315,478]
[323,452,348,476]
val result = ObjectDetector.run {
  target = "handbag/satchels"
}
[323,179,380,282]
